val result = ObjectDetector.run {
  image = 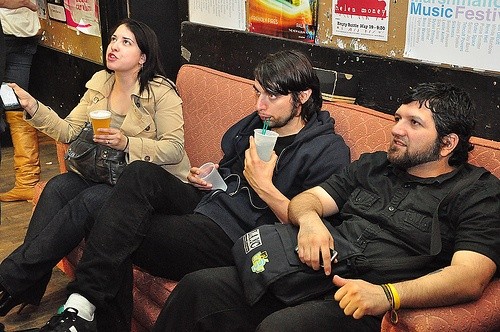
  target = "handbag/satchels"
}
[229,216,374,311]
[63,120,128,187]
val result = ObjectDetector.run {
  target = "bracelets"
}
[381,283,400,311]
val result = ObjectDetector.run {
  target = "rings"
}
[107,140,111,144]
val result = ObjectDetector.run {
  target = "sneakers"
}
[40,305,97,332]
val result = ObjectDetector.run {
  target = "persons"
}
[14,48,352,332]
[0,18,191,318]
[151,81,500,332]
[0,1,75,202]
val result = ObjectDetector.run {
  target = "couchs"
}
[29,64,500,332]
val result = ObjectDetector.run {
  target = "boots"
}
[0,110,43,203]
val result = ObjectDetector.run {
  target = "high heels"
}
[0,278,47,318]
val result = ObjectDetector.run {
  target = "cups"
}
[253,128,279,162]
[90,109,112,135]
[195,162,228,192]
